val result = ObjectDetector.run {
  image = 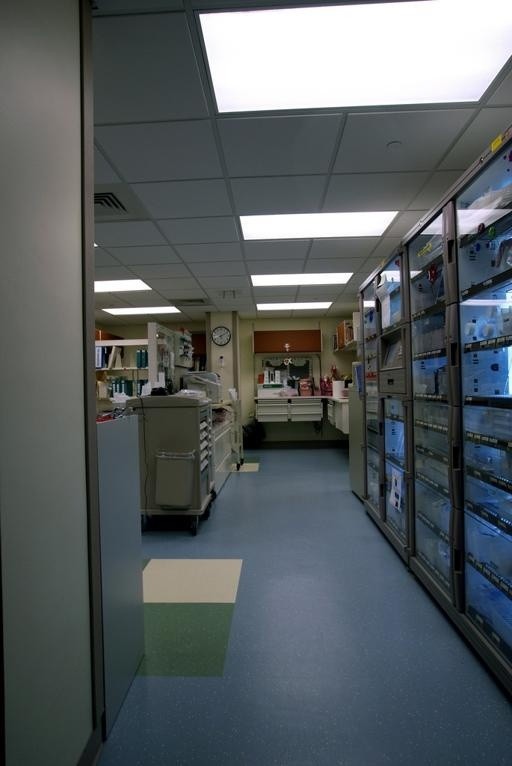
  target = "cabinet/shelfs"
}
[358,119,511,702]
[348,386,367,501]
[253,396,325,423]
[327,396,349,435]
[95,321,194,405]
[123,393,238,537]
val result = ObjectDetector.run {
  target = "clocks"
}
[211,325,231,346]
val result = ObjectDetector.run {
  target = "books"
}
[108,347,122,369]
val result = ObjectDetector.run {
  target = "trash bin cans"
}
[154,448,196,506]
